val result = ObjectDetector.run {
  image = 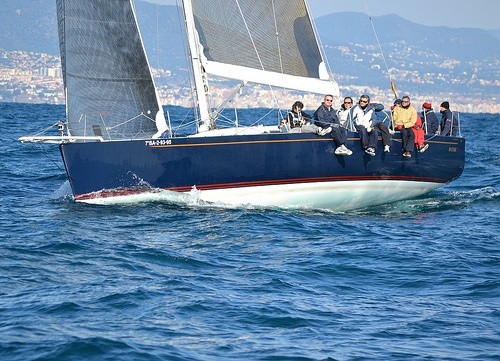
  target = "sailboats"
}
[18,0,468,217]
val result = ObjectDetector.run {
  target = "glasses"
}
[360,100,368,103]
[345,102,351,104]
[402,101,409,103]
[326,99,332,102]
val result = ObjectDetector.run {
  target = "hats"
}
[441,102,449,109]
[423,102,431,109]
[394,99,402,104]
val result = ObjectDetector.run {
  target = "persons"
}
[314,95,353,155]
[370,103,391,152]
[390,96,457,158]
[352,95,379,156]
[335,97,356,133]
[278,101,332,135]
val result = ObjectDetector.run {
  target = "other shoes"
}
[365,148,376,156]
[403,151,412,157]
[319,127,332,136]
[341,144,353,154]
[384,145,390,153]
[334,147,351,155]
[419,144,429,153]
